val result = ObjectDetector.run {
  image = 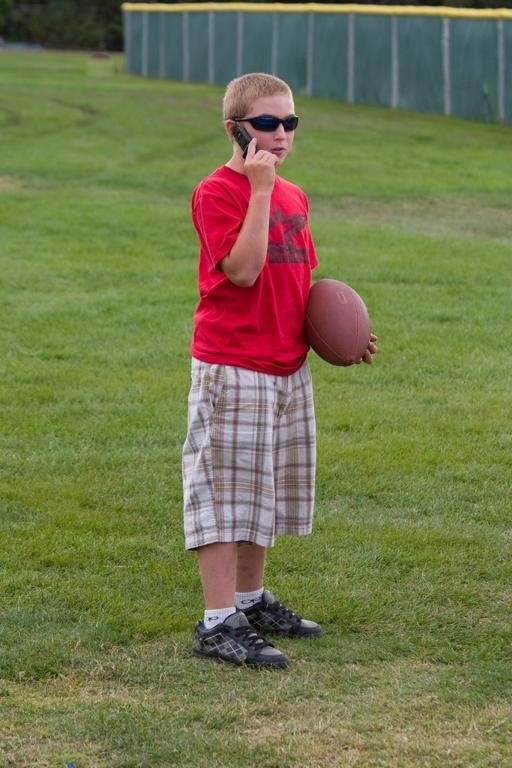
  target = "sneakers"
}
[235,590,322,639]
[192,611,289,669]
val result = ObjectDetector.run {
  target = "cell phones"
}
[228,120,257,159]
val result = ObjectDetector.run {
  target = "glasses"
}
[235,115,298,132]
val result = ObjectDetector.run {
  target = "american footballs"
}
[306,278,372,365]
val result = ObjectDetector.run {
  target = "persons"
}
[181,72,377,668]
